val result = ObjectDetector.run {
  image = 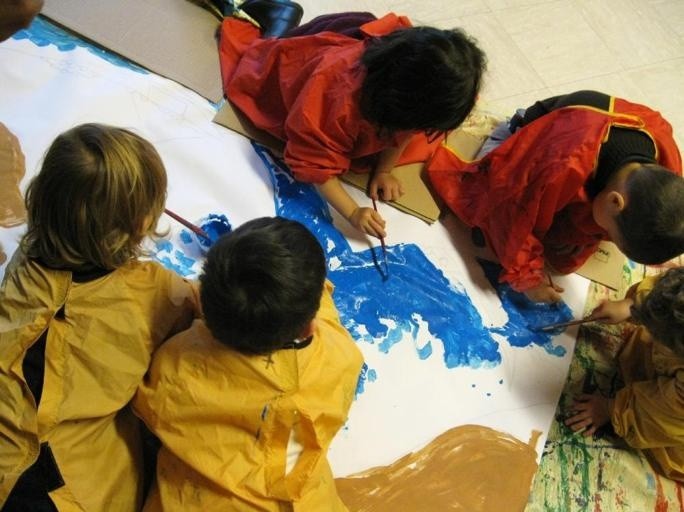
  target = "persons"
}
[470,87,684,306]
[0,121,206,512]
[561,263,684,487]
[215,0,488,240]
[130,216,367,512]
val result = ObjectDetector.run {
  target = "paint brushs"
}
[547,270,560,312]
[539,316,610,331]
[163,206,212,243]
[372,196,391,278]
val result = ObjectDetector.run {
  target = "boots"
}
[208,0,304,37]
